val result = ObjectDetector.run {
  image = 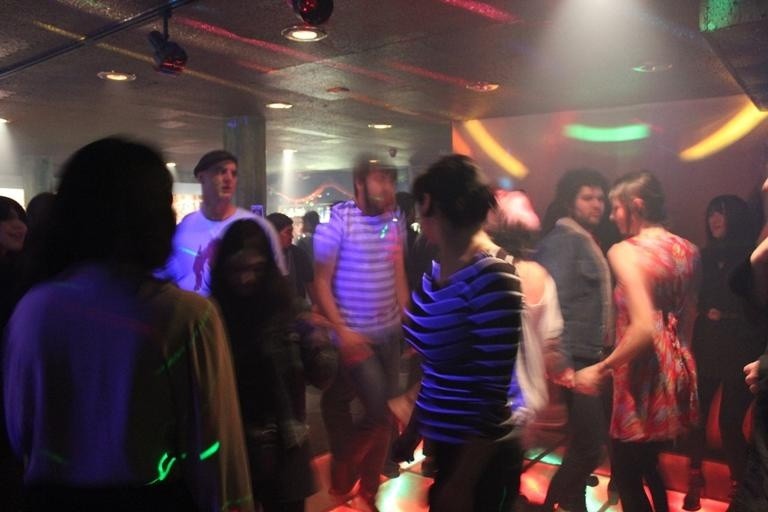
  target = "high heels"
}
[683,479,707,509]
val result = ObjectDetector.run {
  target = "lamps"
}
[144,7,187,84]
[278,3,334,46]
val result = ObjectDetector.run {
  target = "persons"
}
[166,150,287,295]
[1,137,255,512]
[384,193,438,478]
[389,154,526,511]
[294,211,319,253]
[209,218,338,512]
[1,189,72,336]
[684,178,767,511]
[483,169,621,511]
[313,157,409,512]
[268,212,316,310]
[573,171,704,510]
